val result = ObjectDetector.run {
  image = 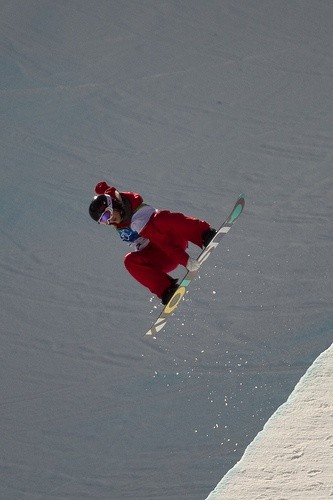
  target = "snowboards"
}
[144,193,246,335]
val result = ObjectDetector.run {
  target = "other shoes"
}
[203,228,218,248]
[162,283,180,305]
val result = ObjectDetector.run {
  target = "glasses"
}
[97,194,114,225]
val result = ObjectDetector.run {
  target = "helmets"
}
[89,195,108,222]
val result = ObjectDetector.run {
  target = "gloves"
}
[186,257,201,272]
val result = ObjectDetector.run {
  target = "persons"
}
[88,182,218,306]
